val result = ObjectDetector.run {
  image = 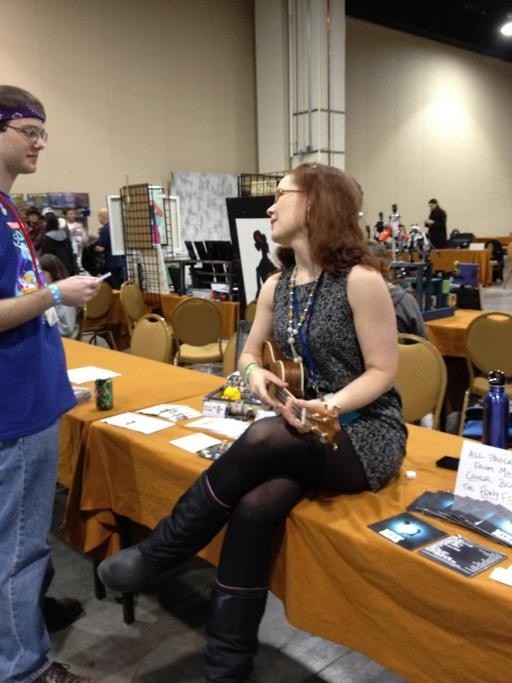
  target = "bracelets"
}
[319,393,330,412]
[241,361,260,387]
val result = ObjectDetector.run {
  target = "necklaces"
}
[286,260,326,363]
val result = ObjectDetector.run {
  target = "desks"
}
[386,247,494,290]
[165,255,236,300]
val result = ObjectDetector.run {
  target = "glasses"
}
[273,187,304,201]
[6,122,50,142]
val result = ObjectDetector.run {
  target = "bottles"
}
[483,367,510,450]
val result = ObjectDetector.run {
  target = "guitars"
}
[261,338,341,451]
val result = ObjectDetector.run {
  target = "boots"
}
[202,579,269,682]
[96,471,231,595]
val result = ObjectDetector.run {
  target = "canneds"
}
[94,376,114,411]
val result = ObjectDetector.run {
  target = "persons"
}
[1,86,106,680]
[96,161,409,681]
[20,203,111,347]
[362,196,463,250]
[366,240,451,433]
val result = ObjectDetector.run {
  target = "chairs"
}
[483,239,505,285]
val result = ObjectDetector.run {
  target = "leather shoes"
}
[41,595,81,634]
[24,660,92,683]
